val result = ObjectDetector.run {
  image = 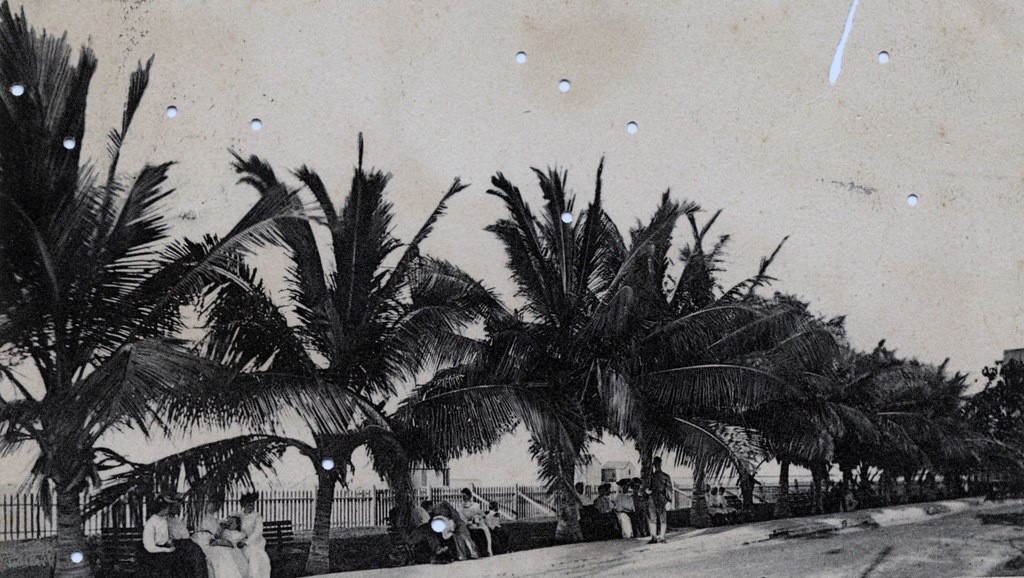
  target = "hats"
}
[155,495,177,507]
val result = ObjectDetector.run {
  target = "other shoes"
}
[436,546,448,554]
[648,538,658,544]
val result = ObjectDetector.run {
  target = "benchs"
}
[383,513,508,565]
[100,521,304,578]
[771,493,832,518]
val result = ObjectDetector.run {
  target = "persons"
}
[143,491,272,578]
[704,484,741,526]
[389,488,499,564]
[573,456,672,543]
